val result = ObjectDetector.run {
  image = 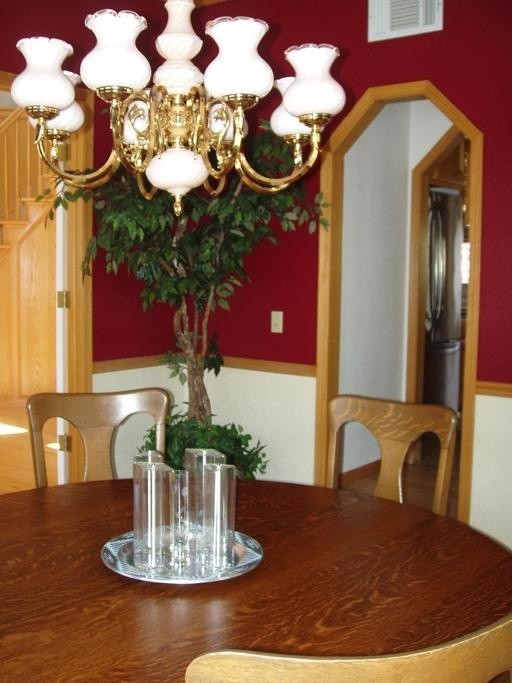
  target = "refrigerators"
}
[422,186,464,413]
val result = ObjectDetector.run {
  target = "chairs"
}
[327,394,459,517]
[26,388,170,488]
[183,615,512,682]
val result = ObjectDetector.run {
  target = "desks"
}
[1,479,511,681]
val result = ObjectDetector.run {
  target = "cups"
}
[132,447,238,575]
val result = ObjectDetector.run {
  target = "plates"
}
[100,520,265,586]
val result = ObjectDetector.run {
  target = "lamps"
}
[11,1,348,216]
